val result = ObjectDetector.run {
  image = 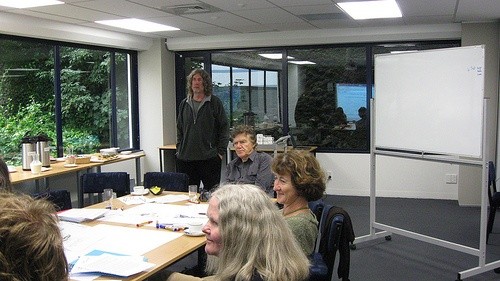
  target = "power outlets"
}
[326,171,333,180]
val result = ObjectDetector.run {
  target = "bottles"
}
[30,154,42,174]
[66,145,74,164]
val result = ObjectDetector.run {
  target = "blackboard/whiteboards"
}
[372,44,487,165]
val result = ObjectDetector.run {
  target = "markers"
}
[136,220,153,227]
[156,218,159,228]
[160,223,188,232]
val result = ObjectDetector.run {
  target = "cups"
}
[189,185,197,201]
[189,221,203,234]
[104,189,113,209]
[133,186,144,193]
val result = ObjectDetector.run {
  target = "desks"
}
[78,150,146,206]
[5,153,105,204]
[159,142,319,174]
[332,122,356,132]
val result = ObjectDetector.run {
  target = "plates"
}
[131,189,149,195]
[187,233,205,236]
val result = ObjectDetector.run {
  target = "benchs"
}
[55,189,283,281]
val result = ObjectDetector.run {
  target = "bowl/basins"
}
[50,159,56,164]
[100,148,120,155]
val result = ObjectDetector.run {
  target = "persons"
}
[334,107,368,140]
[174,68,228,192]
[268,148,327,257]
[0,187,69,281]
[219,125,275,197]
[0,159,12,191]
[150,184,311,281]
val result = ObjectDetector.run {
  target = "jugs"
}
[22,131,53,171]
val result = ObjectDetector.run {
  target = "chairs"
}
[142,170,189,193]
[324,204,351,281]
[78,171,131,207]
[309,197,323,217]
[303,252,329,281]
[34,189,74,209]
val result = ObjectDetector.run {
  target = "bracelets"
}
[283,207,309,216]
